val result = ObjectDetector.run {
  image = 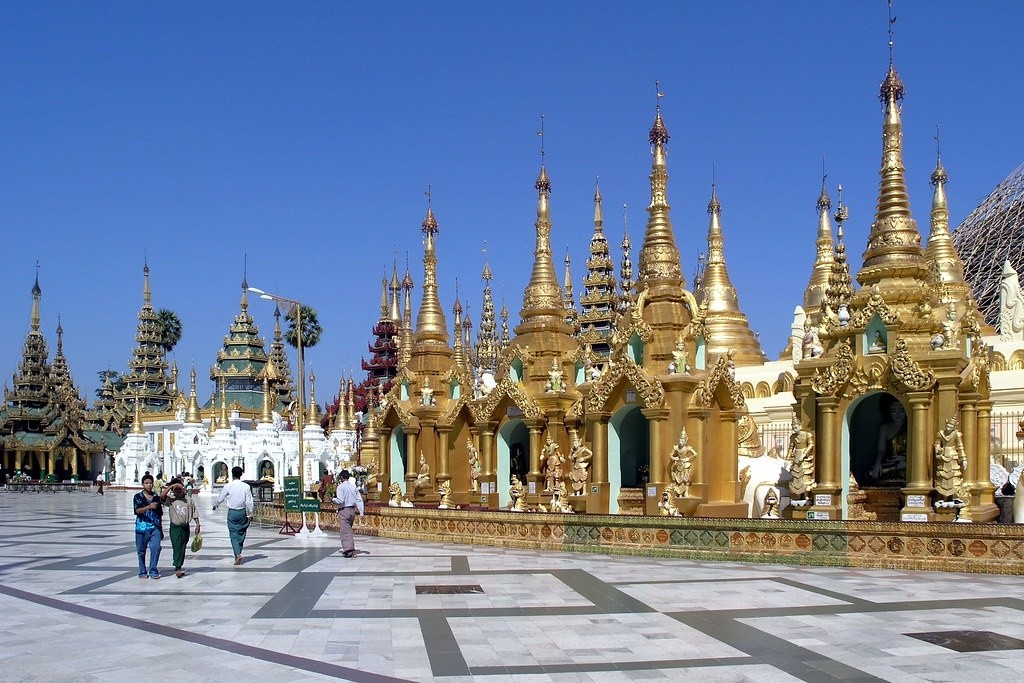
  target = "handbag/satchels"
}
[191,533,203,552]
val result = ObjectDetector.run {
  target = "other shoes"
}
[141,576,148,579]
[343,549,356,558]
[233,555,242,565]
[176,569,182,578]
[153,576,160,579]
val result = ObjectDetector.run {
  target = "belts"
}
[342,506,355,508]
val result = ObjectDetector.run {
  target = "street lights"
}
[247,287,304,496]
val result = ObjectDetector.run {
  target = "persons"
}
[322,469,364,557]
[786,417,817,500]
[973,321,987,357]
[414,450,430,488]
[212,467,253,565]
[934,407,967,497]
[671,334,691,376]
[260,461,274,483]
[216,464,228,483]
[476,363,491,397]
[96,471,104,495]
[668,426,697,498]
[865,396,907,486]
[379,384,385,404]
[584,344,594,382]
[802,315,825,358]
[942,302,962,350]
[871,330,884,347]
[466,437,482,491]
[133,471,200,579]
[540,429,592,497]
[420,376,436,407]
[546,357,566,393]
[510,444,527,485]
[365,457,381,489]
